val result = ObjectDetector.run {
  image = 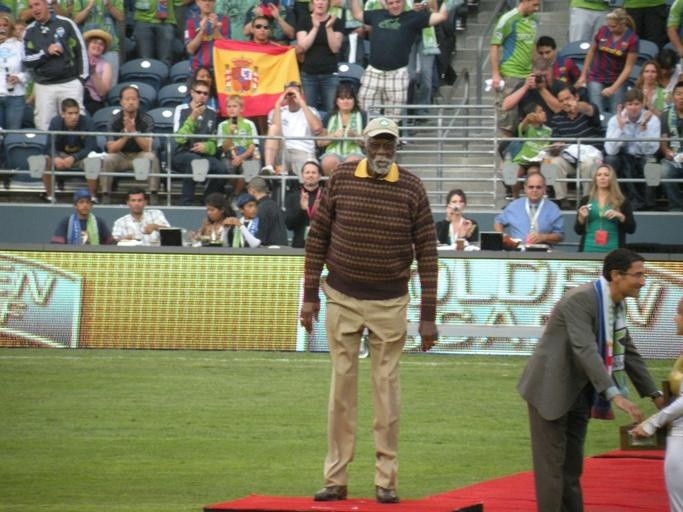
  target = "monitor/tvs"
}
[159,228,182,245]
[480,230,504,250]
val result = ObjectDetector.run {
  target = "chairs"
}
[558,40,660,89]
[0,9,365,190]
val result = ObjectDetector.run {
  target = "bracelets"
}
[649,390,664,400]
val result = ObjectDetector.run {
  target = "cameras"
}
[534,72,542,85]
[451,205,460,214]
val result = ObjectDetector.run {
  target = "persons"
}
[300,118,439,504]
[515,248,672,512]
[1,0,682,255]
[631,296,683,512]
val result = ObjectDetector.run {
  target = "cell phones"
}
[232,115,237,127]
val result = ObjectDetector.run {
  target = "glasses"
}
[525,183,543,190]
[617,270,648,279]
[189,87,208,96]
[256,24,269,29]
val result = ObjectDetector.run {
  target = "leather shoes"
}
[376,485,398,503]
[314,485,347,501]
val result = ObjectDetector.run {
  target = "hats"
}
[250,177,268,194]
[237,193,259,209]
[74,189,92,205]
[363,117,399,140]
[82,29,113,54]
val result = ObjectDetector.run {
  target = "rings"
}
[300,317,304,321]
[432,341,438,344]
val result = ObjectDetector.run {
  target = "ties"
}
[530,204,540,234]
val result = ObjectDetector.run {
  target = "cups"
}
[455,238,465,251]
[7,80,15,93]
